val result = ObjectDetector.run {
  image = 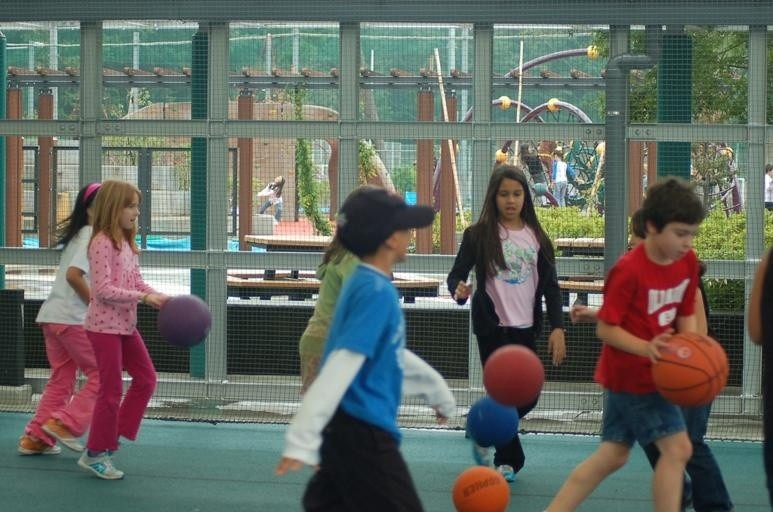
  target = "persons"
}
[19,182,102,455]
[448,165,566,481]
[277,189,456,512]
[300,235,360,395]
[77,180,171,479]
[258,176,285,220]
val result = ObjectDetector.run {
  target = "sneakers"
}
[39,417,86,454]
[15,434,63,456]
[77,446,125,481]
[496,464,517,483]
[473,440,498,468]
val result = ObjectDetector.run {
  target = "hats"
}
[336,187,435,256]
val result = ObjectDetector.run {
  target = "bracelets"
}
[141,292,151,305]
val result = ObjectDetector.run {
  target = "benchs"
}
[226,235,439,303]
[553,237,605,306]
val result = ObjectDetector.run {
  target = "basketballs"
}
[467,399,518,447]
[484,345,544,406]
[157,295,212,346]
[452,466,509,512]
[650,332,728,406]
